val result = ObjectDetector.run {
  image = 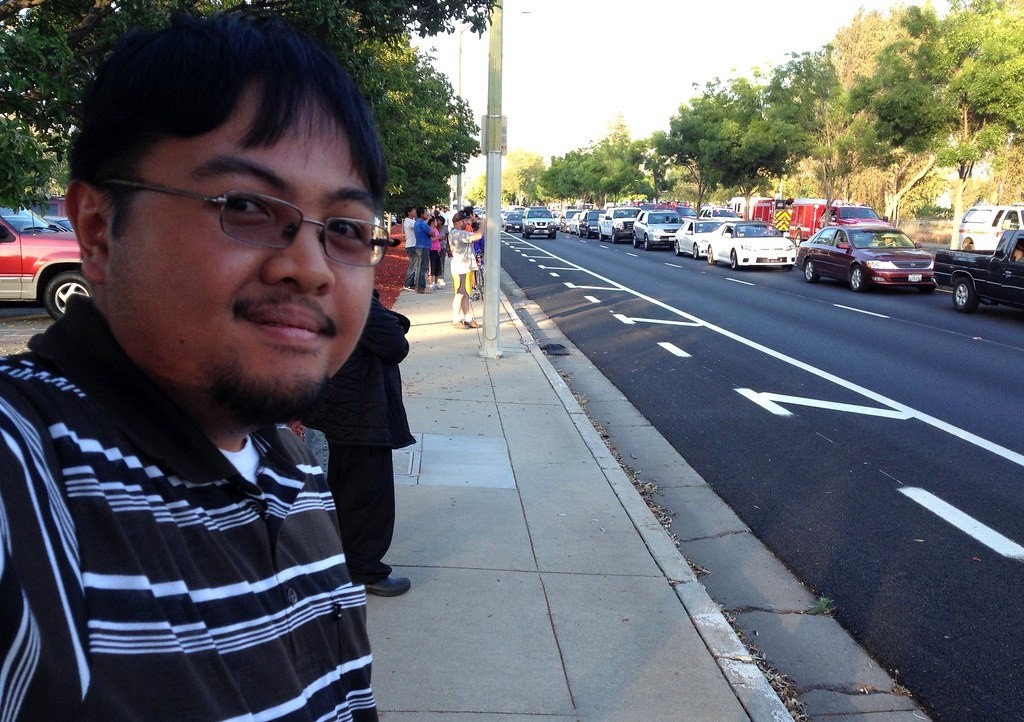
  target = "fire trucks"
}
[752,198,893,248]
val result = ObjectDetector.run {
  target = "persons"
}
[0,12,399,721]
[296,291,413,596]
[403,199,448,293]
[878,238,893,247]
[451,199,488,333]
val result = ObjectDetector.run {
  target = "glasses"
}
[102,177,400,267]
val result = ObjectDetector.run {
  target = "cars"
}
[643,203,697,220]
[41,214,75,233]
[503,213,523,233]
[674,220,727,260]
[568,213,582,236]
[796,224,936,292]
[461,205,486,216]
[501,204,594,234]
[707,222,798,271]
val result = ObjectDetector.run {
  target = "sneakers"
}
[464,320,482,328]
[453,321,470,330]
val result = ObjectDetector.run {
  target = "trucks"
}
[726,197,770,222]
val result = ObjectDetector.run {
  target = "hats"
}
[452,212,472,223]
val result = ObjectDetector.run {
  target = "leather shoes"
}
[366,576,411,597]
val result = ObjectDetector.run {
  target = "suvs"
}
[578,209,606,239]
[957,204,1023,256]
[0,215,93,323]
[1,203,57,233]
[521,206,557,239]
[632,209,685,251]
[699,208,745,223]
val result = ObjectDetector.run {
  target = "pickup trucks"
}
[597,207,642,245]
[934,229,1024,313]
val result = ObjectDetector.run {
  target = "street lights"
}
[457,7,533,219]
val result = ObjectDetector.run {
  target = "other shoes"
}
[403,285,415,291]
[430,284,442,292]
[417,288,431,294]
